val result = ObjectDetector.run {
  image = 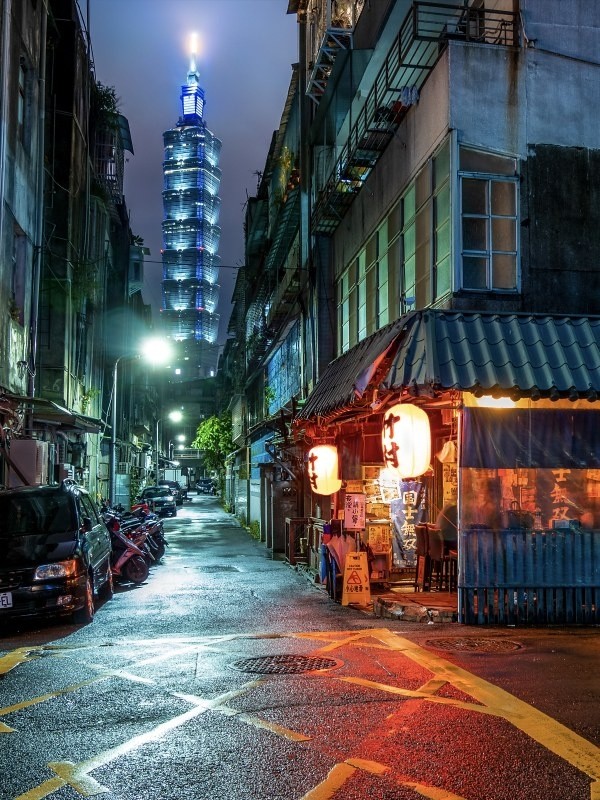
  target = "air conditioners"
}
[9,439,48,486]
[438,19,478,56]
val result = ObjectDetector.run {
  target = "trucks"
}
[163,468,191,498]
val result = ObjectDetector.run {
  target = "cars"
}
[159,479,184,506]
[136,485,177,517]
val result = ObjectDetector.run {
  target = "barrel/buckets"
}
[507,501,532,531]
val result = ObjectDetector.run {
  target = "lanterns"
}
[381,403,430,482]
[308,445,341,496]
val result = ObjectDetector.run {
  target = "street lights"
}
[156,410,182,486]
[168,434,185,467]
[109,337,169,510]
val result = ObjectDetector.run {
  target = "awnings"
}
[5,393,112,434]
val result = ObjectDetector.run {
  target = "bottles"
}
[317,507,321,519]
[533,511,542,530]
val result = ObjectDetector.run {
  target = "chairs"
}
[413,524,456,593]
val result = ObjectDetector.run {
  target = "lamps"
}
[403,297,415,311]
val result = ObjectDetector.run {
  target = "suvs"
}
[0,478,114,624]
[195,478,215,495]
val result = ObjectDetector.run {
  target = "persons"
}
[436,486,473,550]
[479,477,504,529]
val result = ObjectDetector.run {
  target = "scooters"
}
[96,498,169,584]
